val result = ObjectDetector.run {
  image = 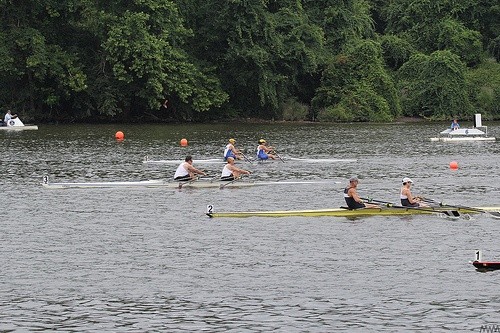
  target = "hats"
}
[349,176,358,181]
[260,139,265,142]
[402,177,413,183]
[229,139,235,142]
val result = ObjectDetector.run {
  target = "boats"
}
[0,116,38,131]
[473,250,500,270]
[42,171,341,189]
[430,114,496,142]
[141,150,358,165]
[204,197,500,218]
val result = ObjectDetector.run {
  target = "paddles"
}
[273,150,286,163]
[359,197,395,205]
[240,149,252,164]
[218,174,247,190]
[178,174,209,190]
[412,197,484,214]
[363,201,460,218]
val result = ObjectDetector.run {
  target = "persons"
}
[174,156,206,180]
[224,139,243,160]
[257,139,280,160]
[344,176,378,209]
[4,110,17,123]
[400,178,429,208]
[451,120,459,130]
[222,157,249,180]
